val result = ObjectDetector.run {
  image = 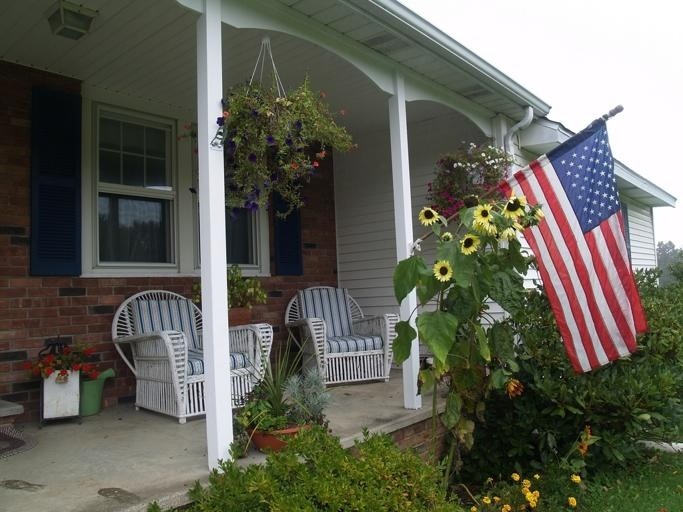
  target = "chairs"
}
[106,284,276,426]
[282,284,406,391]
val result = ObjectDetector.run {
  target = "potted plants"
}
[227,325,335,462]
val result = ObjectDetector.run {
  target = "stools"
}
[0,397,42,462]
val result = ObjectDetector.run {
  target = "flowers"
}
[187,261,271,314]
[21,337,101,385]
[209,62,361,222]
[421,141,514,224]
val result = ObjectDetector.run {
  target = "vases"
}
[225,303,255,329]
[37,367,80,420]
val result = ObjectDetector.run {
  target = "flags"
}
[500,114,648,373]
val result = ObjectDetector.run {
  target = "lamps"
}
[46,0,99,42]
